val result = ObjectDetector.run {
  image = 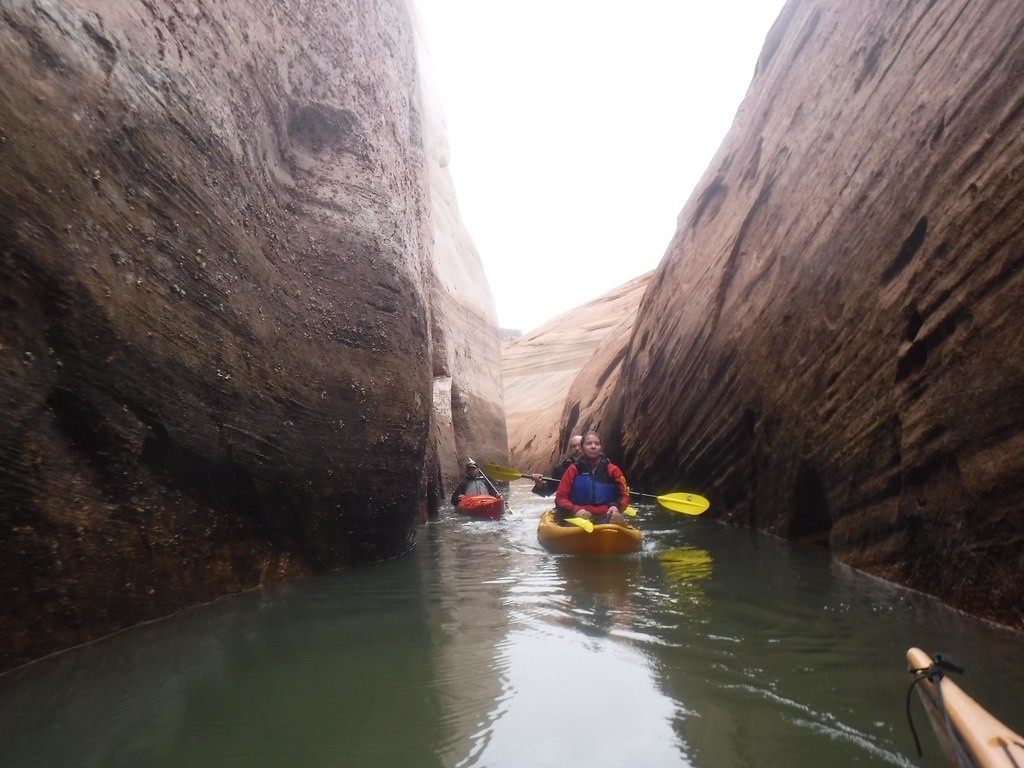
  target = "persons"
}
[553,430,631,528]
[451,461,503,507]
[530,435,626,521]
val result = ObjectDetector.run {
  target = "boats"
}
[538,505,643,555]
[903,646,1024,768]
[457,492,506,519]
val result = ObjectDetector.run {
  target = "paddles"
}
[563,506,637,533]
[467,456,515,516]
[487,463,711,515]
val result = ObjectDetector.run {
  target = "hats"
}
[465,462,479,469]
